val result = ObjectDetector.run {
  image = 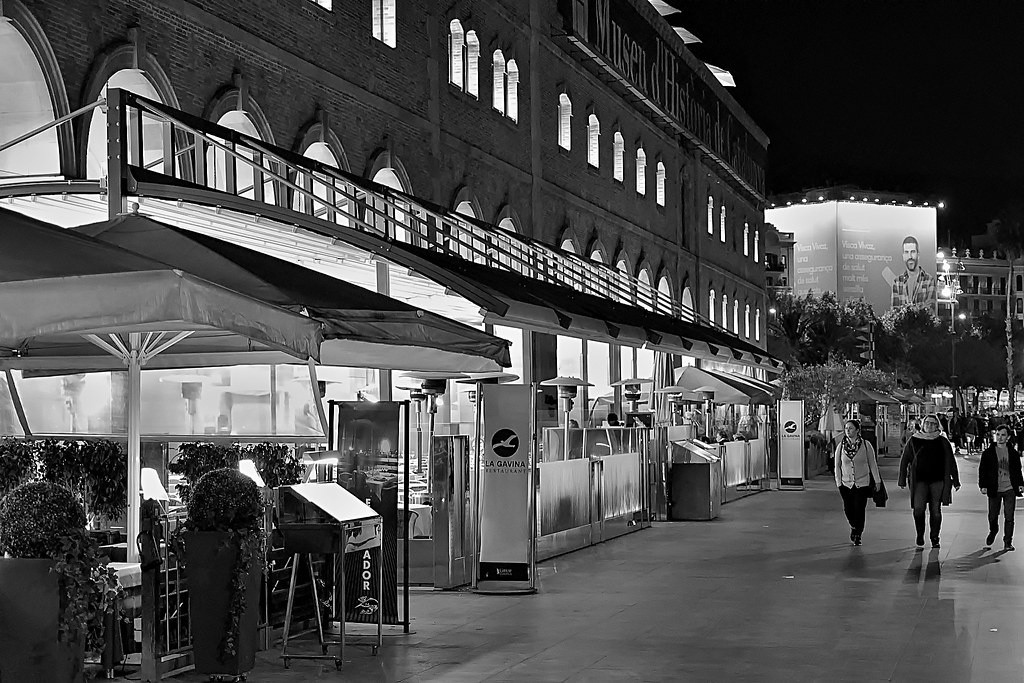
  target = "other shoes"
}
[915,534,926,547]
[931,537,941,549]
[1004,540,1015,552]
[986,532,997,546]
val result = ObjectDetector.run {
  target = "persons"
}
[569,412,620,429]
[897,414,961,550]
[834,420,881,546]
[891,236,937,313]
[936,410,1024,457]
[978,424,1024,550]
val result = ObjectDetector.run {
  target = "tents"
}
[843,386,902,419]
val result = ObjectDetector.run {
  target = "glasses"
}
[925,421,936,425]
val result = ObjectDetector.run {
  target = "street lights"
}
[934,251,965,426]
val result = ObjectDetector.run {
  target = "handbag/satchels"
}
[867,473,876,498]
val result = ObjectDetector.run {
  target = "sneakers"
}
[850,527,856,542]
[854,529,863,546]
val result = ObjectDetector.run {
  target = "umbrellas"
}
[891,388,929,428]
[0,202,512,564]
[673,362,782,443]
